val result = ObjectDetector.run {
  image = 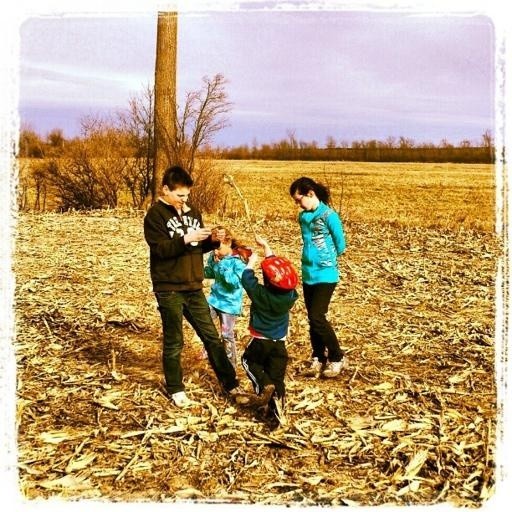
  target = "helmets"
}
[261,256,298,291]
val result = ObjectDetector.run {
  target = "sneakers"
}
[304,357,325,376]
[228,385,250,404]
[167,391,191,408]
[322,355,346,377]
[254,384,275,405]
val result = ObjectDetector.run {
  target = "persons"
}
[242,234,300,430]
[289,177,346,377]
[196,226,252,380]
[143,164,250,408]
